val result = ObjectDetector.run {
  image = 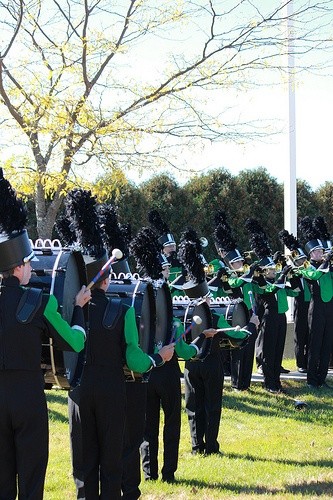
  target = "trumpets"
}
[202,263,214,275]
[289,261,311,273]
[254,262,282,274]
[225,263,252,274]
[198,236,210,247]
[320,252,332,257]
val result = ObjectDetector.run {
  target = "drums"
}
[172,297,215,362]
[151,281,174,348]
[23,247,90,392]
[210,297,251,351]
[104,279,156,383]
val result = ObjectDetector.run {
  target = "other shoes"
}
[280,366,290,373]
[265,386,289,394]
[223,367,231,376]
[231,386,254,394]
[305,383,332,391]
[297,367,307,373]
[257,365,264,374]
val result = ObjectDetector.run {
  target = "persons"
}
[0,229,93,500]
[140,271,217,484]
[66,249,175,500]
[58,234,333,394]
[183,277,261,457]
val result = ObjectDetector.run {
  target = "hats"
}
[245,218,275,269]
[0,168,35,273]
[149,209,176,247]
[313,216,333,250]
[143,227,171,268]
[178,231,210,299]
[213,223,245,266]
[96,203,131,280]
[279,229,308,262]
[296,210,324,255]
[68,188,112,283]
